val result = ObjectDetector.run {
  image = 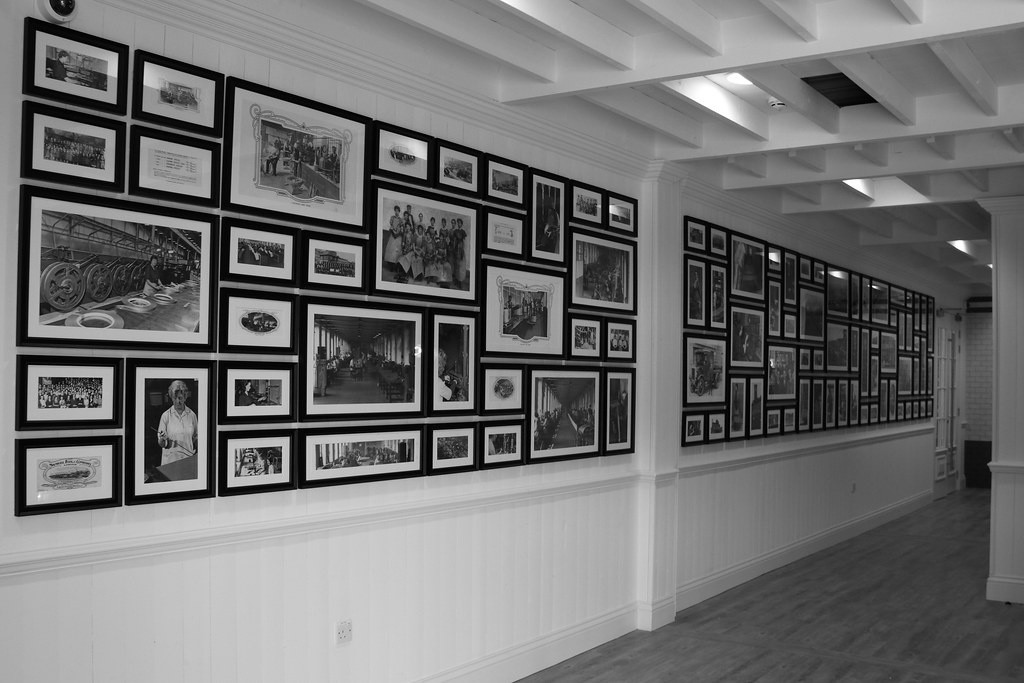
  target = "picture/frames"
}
[681,214,935,448]
[12,16,638,518]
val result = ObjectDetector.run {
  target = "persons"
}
[239,240,284,266]
[384,205,467,289]
[54,50,88,86]
[584,263,624,302]
[537,198,560,253]
[489,434,498,455]
[438,350,457,404]
[576,195,597,216]
[236,379,261,406]
[143,255,165,298]
[575,326,596,350]
[266,134,340,184]
[158,381,198,467]
[39,378,102,408]
[611,329,628,352]
[267,456,276,474]
[44,134,105,169]
